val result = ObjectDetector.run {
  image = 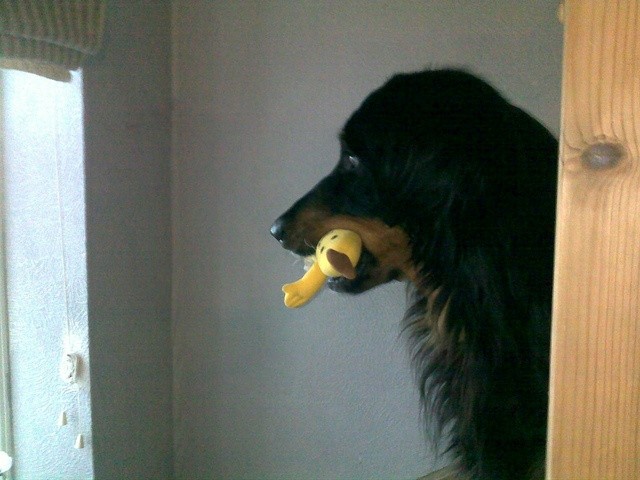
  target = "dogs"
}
[269,62,559,480]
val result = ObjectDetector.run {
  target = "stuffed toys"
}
[282,227,363,310]
